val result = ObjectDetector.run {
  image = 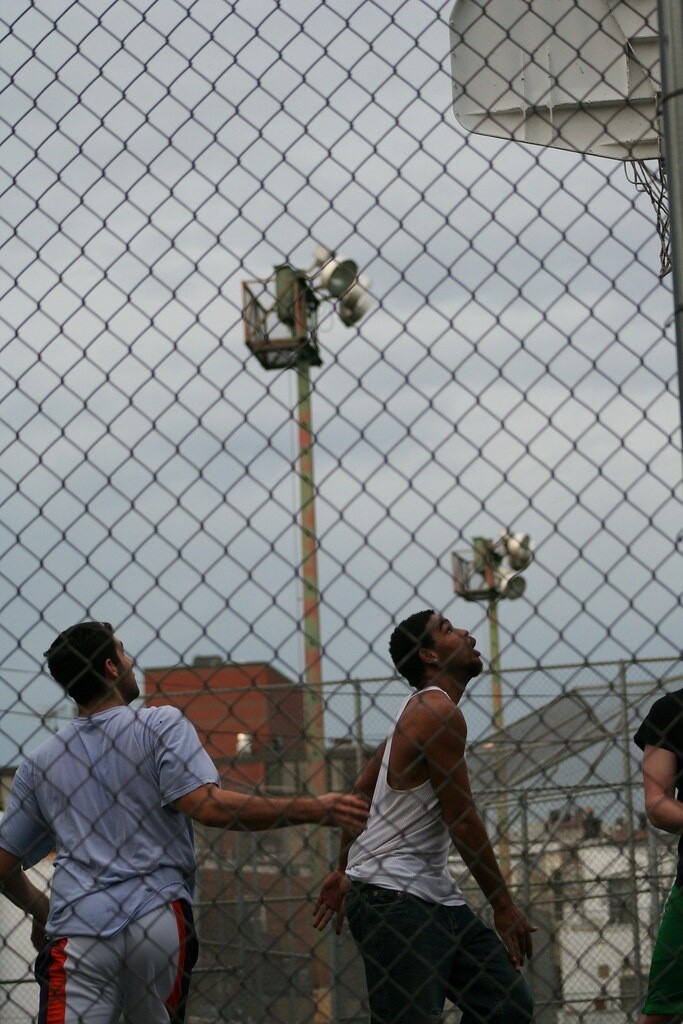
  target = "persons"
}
[311,608,538,1024]
[0,622,372,1024]
[634,689,683,1024]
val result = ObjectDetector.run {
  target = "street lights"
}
[294,256,382,1023]
[478,532,538,894]
